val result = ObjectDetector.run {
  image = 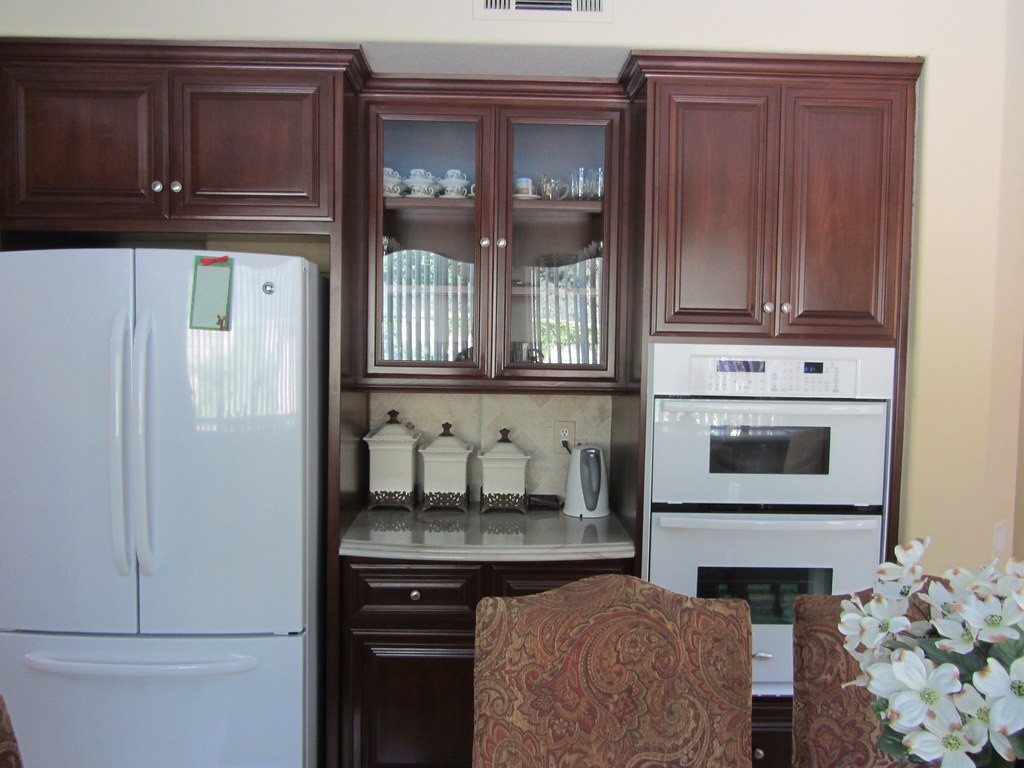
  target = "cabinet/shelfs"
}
[351,69,628,397]
[1,36,372,233]
[328,558,644,768]
[618,50,927,347]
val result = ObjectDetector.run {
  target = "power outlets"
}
[554,422,576,453]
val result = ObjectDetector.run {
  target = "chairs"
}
[475,575,751,768]
[792,575,953,768]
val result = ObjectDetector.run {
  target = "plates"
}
[403,179,433,186]
[440,195,467,198]
[383,178,401,185]
[439,178,469,187]
[467,193,476,199]
[405,194,434,198]
[383,193,401,197]
[513,194,542,200]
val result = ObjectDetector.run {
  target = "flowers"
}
[840,538,1024,768]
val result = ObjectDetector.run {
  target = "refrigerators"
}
[0,249,323,767]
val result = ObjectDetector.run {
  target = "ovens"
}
[642,342,906,768]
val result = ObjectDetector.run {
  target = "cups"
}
[516,178,534,195]
[410,169,431,179]
[446,169,466,180]
[549,176,569,200]
[569,166,604,201]
[411,184,433,196]
[383,168,399,178]
[445,186,467,196]
[383,185,400,193]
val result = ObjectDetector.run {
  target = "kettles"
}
[562,445,610,518]
